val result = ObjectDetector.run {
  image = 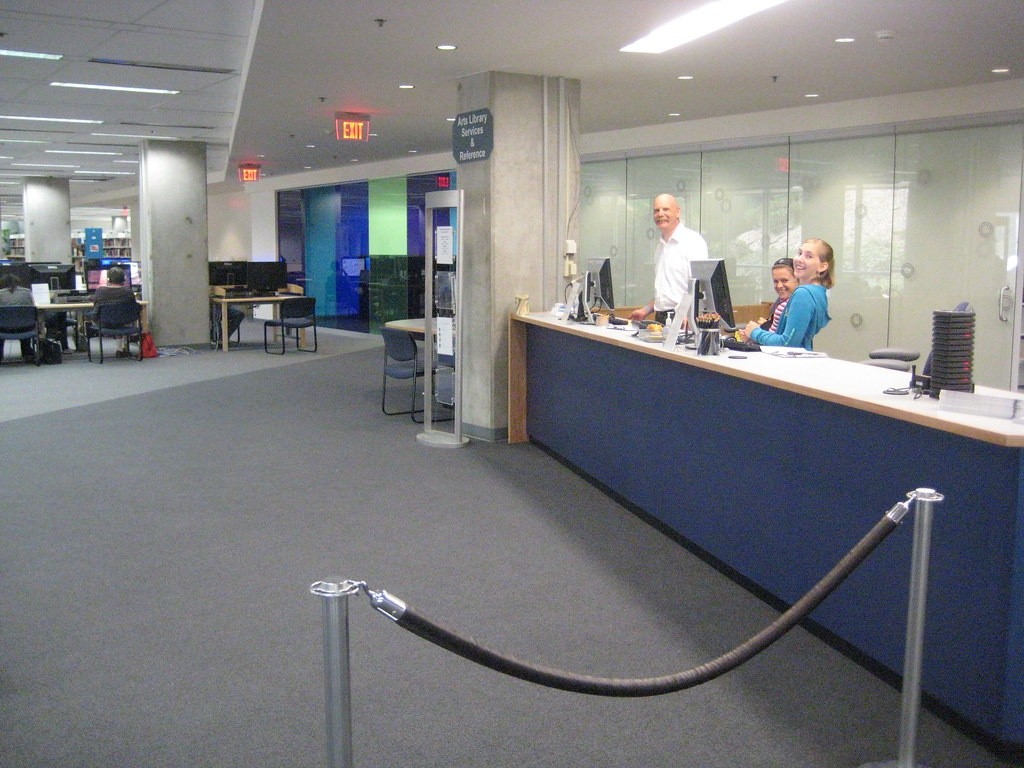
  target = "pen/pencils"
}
[696,313,720,356]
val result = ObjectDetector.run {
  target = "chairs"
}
[265,297,318,355]
[377,326,455,424]
[0,305,46,366]
[84,301,143,365]
[65,310,79,352]
[861,301,974,376]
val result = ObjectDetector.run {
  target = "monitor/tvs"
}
[0,260,75,293]
[248,262,287,290]
[208,261,247,286]
[85,257,142,292]
[685,259,735,350]
[580,257,614,325]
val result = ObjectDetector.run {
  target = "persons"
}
[218,307,245,347]
[737,238,835,352]
[0,273,37,362]
[91,266,137,358]
[626,193,708,331]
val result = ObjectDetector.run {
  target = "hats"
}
[774,257,793,267]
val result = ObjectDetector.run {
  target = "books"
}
[72,238,131,272]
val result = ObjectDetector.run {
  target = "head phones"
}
[106,266,126,283]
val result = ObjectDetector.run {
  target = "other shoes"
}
[62,349,73,353]
[116,349,132,358]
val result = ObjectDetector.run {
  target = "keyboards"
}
[719,339,761,352]
[592,314,628,325]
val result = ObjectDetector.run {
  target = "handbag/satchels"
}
[137,334,156,357]
[72,333,88,351]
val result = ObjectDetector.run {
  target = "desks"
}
[35,299,149,356]
[210,281,305,352]
[386,318,457,394]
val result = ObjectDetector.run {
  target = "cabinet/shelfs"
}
[5,237,132,273]
